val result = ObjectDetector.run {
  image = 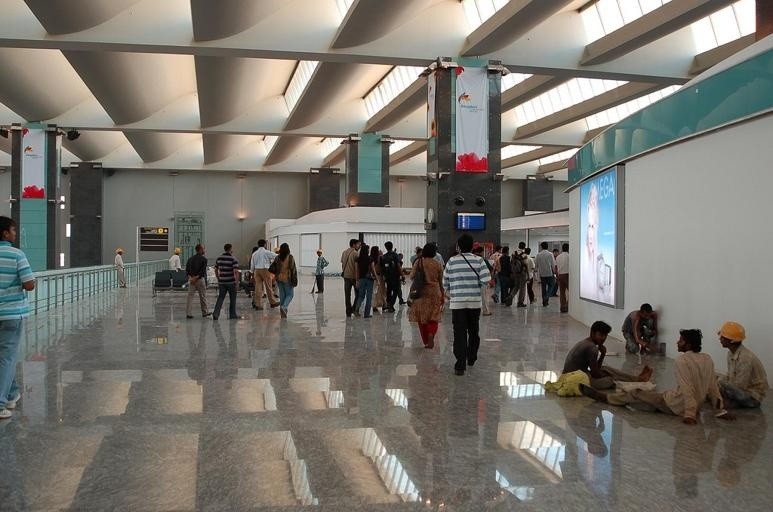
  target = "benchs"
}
[240,270,255,298]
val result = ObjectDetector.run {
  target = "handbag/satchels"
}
[407,270,427,300]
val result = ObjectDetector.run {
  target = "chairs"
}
[152,269,207,298]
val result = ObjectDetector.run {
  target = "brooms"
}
[309,278,317,295]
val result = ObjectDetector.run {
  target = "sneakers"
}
[5,391,21,409]
[483,293,568,318]
[341,300,407,320]
[186,302,288,320]
[423,334,435,349]
[453,358,477,375]
[0,408,12,418]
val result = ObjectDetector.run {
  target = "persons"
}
[621,303,660,353]
[315,250,329,293]
[115,248,127,288]
[577,328,737,425]
[715,321,770,407]
[169,248,181,272]
[212,244,243,320]
[0,215,35,418]
[186,244,212,318]
[562,321,653,389]
[249,239,298,318]
[579,183,611,303]
[341,239,569,375]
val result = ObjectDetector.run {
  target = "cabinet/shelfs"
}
[138,227,169,251]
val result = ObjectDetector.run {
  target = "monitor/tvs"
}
[456,211,487,231]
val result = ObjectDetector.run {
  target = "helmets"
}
[175,247,180,254]
[273,248,280,253]
[115,248,127,254]
[717,321,747,343]
[316,249,323,255]
